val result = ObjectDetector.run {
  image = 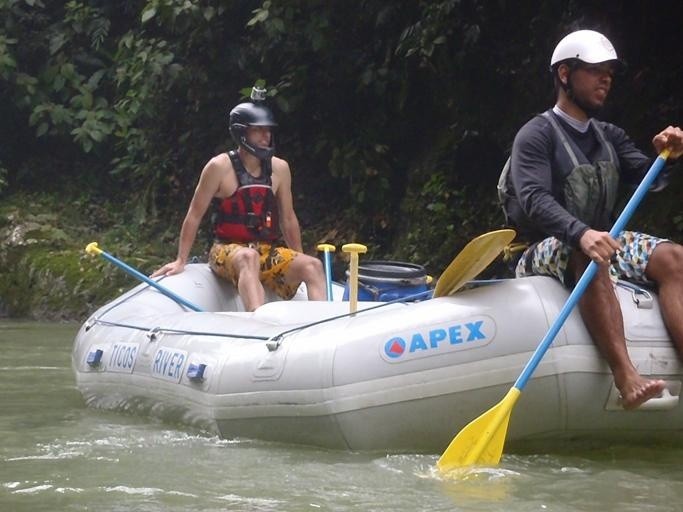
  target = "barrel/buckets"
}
[342,261,433,303]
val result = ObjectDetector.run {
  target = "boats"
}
[70,263,682,453]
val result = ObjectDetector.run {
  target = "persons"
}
[142,101,327,312]
[492,27,682,413]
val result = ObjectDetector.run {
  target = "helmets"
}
[549,28,629,78]
[227,100,279,162]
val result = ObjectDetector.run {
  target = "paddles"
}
[438,148,670,471]
[431,228,516,298]
